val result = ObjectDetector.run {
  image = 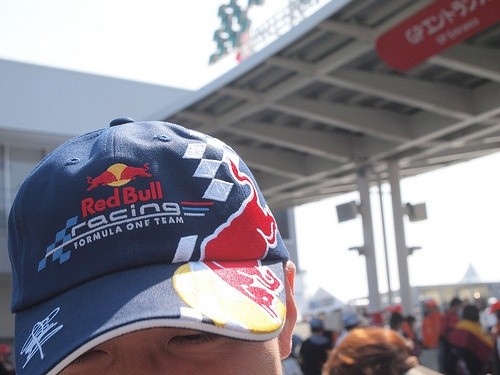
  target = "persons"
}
[7,116,298,375]
[0,345,16,375]
[280,294,500,375]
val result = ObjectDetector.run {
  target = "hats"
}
[342,314,360,328]
[311,319,323,329]
[8,118,290,375]
